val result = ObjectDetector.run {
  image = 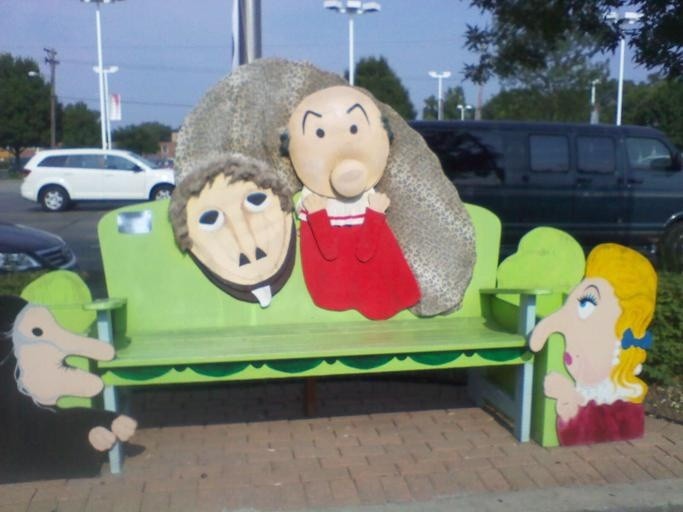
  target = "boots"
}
[21,147,175,210]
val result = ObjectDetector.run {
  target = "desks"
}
[585,77,603,124]
[80,0,122,148]
[322,0,382,85]
[94,64,118,149]
[427,70,453,120]
[588,10,645,124]
[456,104,474,121]
[23,69,56,150]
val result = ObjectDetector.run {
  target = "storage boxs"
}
[79,183,552,475]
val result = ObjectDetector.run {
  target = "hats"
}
[1,223,78,295]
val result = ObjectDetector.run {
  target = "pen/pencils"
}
[405,121,682,274]
